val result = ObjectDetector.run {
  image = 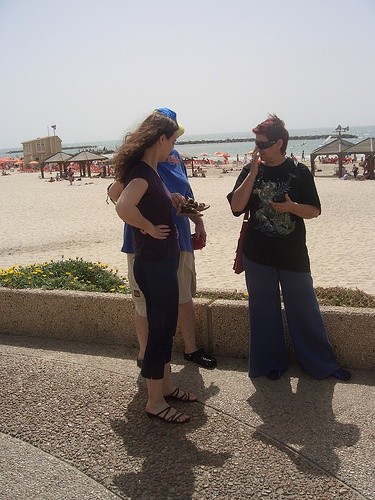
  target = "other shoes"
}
[333,367,352,380]
[265,370,280,380]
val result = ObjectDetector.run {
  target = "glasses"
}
[255,139,277,149]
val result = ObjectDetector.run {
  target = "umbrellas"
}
[199,153,209,156]
[214,152,230,158]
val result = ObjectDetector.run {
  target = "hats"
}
[154,107,184,136]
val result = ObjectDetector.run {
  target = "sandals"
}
[176,196,211,217]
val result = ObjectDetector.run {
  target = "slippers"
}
[164,387,197,402]
[136,359,171,374]
[184,348,217,368]
[147,405,189,423]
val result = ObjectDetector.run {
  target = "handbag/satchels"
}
[233,217,250,274]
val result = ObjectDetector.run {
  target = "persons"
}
[291,153,297,161]
[120,108,215,370]
[244,154,247,165]
[193,165,207,177]
[319,157,353,164]
[353,155,375,178]
[227,117,353,381]
[107,112,217,424]
[302,150,305,161]
[50,165,74,185]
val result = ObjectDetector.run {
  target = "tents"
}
[41,151,108,180]
[339,137,375,178]
[311,139,355,176]
